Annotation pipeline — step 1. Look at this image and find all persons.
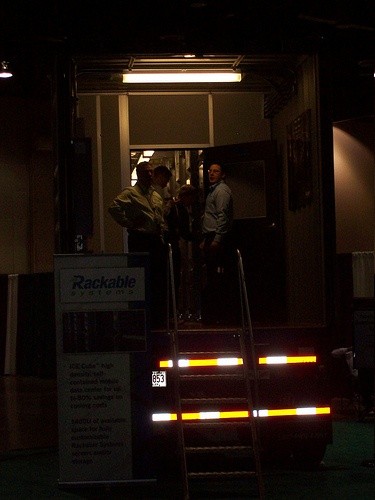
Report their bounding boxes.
[109,161,167,332]
[155,166,206,321]
[200,163,234,324]
